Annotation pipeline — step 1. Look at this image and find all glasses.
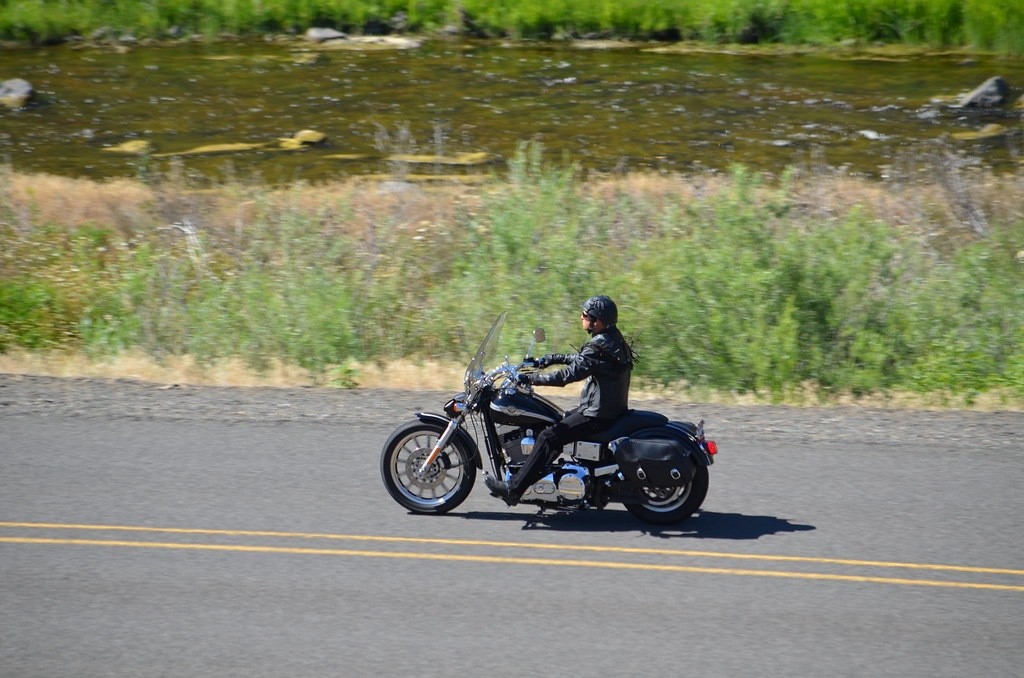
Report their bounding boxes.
[583,312,589,318]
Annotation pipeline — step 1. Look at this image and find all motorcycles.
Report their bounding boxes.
[380,310,719,525]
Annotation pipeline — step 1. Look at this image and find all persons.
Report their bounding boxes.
[483,296,632,505]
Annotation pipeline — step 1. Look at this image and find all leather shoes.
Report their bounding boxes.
[484,474,520,506]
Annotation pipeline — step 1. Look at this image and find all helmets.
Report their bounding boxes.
[583,295,617,324]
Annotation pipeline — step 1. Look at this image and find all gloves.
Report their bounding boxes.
[541,354,553,366]
[514,373,529,386]
